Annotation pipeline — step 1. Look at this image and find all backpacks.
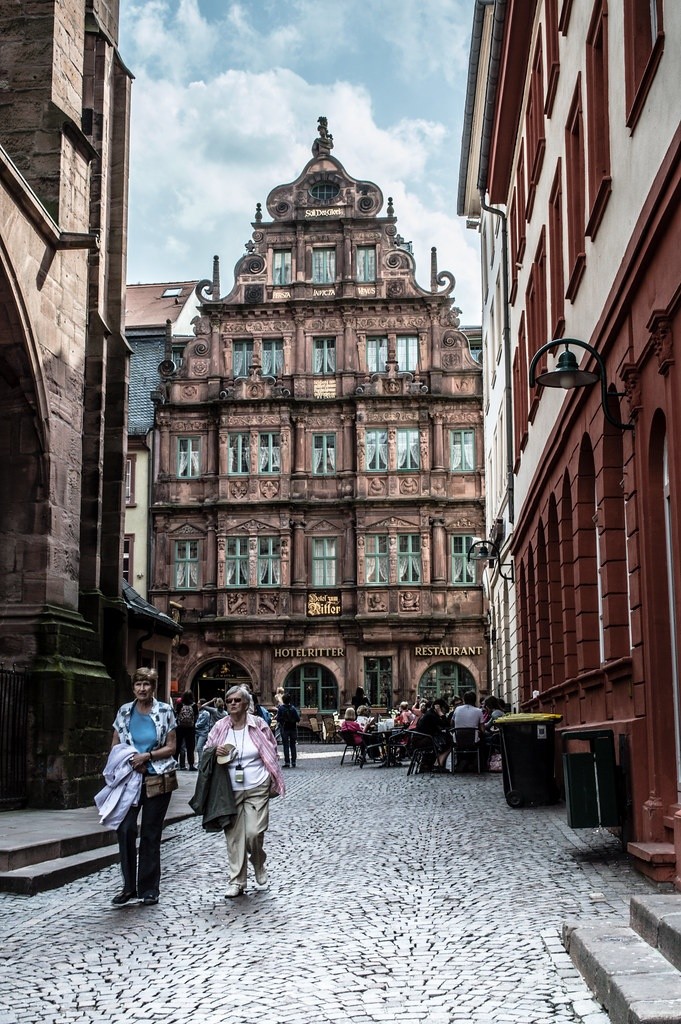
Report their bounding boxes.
[177,702,195,728]
[282,706,297,730]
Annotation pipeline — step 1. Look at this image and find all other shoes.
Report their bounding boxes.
[282,765,290,768]
[225,884,243,898]
[189,767,198,771]
[256,866,267,885]
[432,764,449,773]
[113,892,137,904]
[292,764,296,767]
[180,768,187,770]
[145,896,158,905]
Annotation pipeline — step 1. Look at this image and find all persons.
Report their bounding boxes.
[351,686,372,718]
[111,668,177,906]
[170,683,301,771]
[341,691,506,773]
[202,686,285,898]
[311,125,334,157]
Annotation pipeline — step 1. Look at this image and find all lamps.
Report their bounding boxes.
[467,541,514,584]
[529,337,634,431]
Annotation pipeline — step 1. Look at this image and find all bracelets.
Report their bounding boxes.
[146,751,152,762]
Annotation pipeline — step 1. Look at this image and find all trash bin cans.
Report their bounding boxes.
[493,713,564,809]
[560,728,622,829]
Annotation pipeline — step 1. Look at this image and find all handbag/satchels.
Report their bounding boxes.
[145,770,178,797]
[489,754,502,772]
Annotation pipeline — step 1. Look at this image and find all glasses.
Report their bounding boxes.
[226,698,241,703]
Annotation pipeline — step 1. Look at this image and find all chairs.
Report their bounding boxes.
[336,729,366,766]
[404,729,443,776]
[357,731,387,769]
[448,727,484,776]
[388,729,411,768]
